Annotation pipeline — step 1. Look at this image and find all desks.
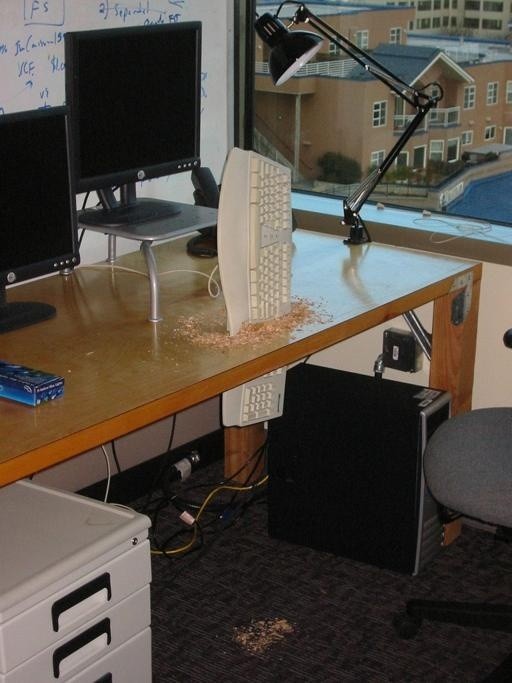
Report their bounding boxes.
[0,229,482,546]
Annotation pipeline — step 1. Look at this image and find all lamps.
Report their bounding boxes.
[254,0,444,244]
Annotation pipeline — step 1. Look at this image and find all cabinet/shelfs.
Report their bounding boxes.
[0,480,153,683]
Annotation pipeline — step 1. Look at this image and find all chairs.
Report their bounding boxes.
[391,329,512,683]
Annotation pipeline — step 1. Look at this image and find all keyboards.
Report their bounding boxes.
[216,146,293,431]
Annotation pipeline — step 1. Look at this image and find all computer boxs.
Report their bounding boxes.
[268,362,454,581]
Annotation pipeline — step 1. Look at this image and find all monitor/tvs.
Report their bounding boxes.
[1,103,82,337]
[61,19,203,229]
